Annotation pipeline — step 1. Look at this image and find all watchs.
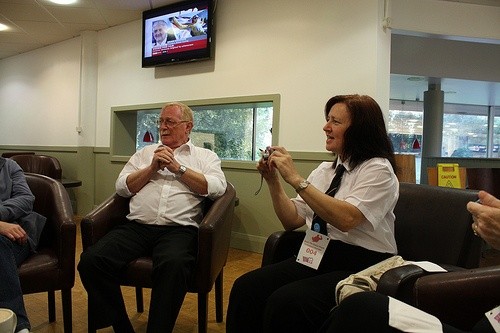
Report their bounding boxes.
[296,180,310,193]
[177,165,186,176]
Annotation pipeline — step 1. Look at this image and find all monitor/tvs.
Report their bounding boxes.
[141,0,215,68]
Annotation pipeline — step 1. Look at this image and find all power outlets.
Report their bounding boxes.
[76,127,82,132]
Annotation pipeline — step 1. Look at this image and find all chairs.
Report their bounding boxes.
[259,181,488,333]
[15,171,77,333]
[77,180,237,333]
[10,154,63,180]
[412,264,500,333]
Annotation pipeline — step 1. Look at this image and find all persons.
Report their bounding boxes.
[76,102,227,333]
[170,15,206,37]
[226,96,399,333]
[152,21,176,45]
[467,191,500,249]
[0,156,35,333]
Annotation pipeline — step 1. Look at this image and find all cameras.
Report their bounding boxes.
[262,148,275,160]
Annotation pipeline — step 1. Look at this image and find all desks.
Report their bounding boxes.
[54,178,82,188]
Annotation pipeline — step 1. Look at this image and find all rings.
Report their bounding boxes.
[474,226,478,235]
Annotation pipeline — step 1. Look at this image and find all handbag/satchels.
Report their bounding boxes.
[335,256,405,306]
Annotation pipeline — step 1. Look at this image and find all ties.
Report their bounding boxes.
[311,164,345,236]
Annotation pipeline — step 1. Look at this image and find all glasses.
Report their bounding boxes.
[156,119,189,129]
